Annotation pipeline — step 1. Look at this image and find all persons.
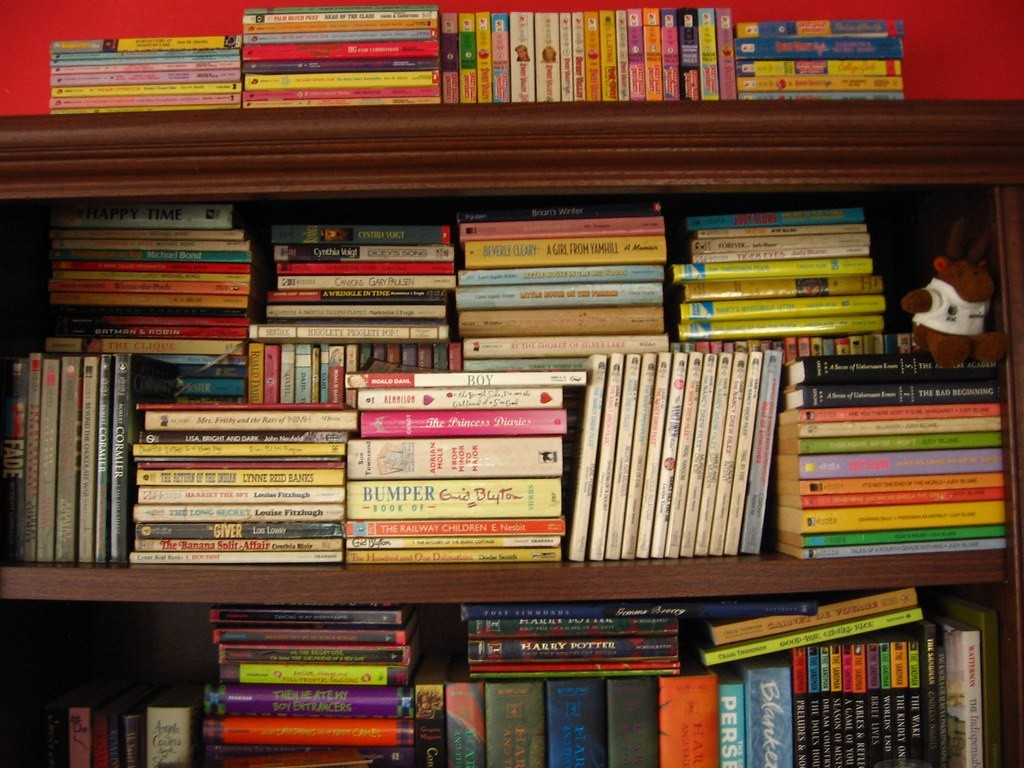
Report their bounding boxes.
[515,45,530,62]
[540,46,556,63]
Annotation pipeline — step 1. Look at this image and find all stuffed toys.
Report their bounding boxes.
[901,216,1010,368]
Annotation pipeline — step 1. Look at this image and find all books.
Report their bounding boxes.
[0,200,1010,565]
[40,585,1003,768]
[440,7,737,104]
[733,19,905,99]
[50,35,243,114]
[242,4,443,108]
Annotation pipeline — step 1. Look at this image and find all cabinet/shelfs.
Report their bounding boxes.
[1,99,1024,767]
